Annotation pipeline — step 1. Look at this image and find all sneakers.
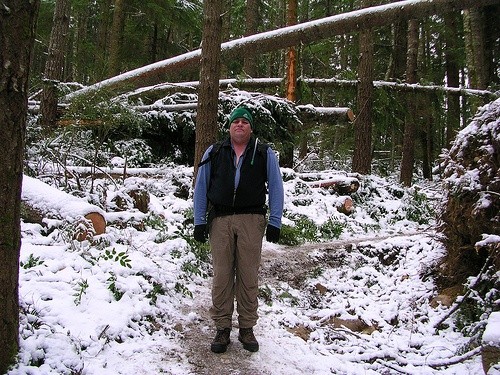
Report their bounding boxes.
[238,328,259,352]
[211,330,230,353]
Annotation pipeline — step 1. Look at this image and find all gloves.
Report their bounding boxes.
[266,224,280,243]
[193,224,208,243]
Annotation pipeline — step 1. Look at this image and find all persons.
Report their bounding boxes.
[191,107,284,352]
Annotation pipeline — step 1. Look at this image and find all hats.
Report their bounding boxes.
[229,108,253,129]
